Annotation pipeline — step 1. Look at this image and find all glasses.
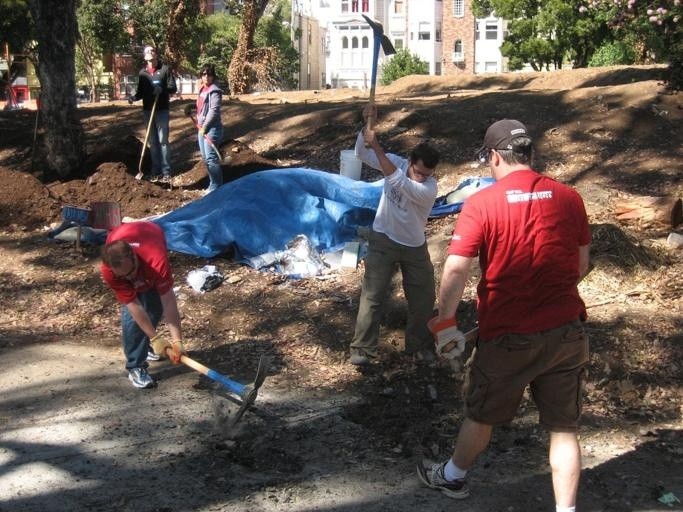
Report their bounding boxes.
[477,149,492,162]
[412,165,435,179]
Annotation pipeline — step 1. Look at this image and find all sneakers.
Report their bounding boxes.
[126,366,155,389]
[145,351,161,362]
[415,458,474,500]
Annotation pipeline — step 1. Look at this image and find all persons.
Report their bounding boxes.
[129,45,177,181]
[101,220,186,388]
[350,102,436,366]
[197,64,225,197]
[416,119,589,511]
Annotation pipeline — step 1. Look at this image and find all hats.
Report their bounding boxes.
[475,118,530,155]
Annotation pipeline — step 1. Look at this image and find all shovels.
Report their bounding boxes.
[135,92,159,180]
[188,113,232,165]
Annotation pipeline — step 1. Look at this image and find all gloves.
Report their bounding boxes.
[171,340,185,368]
[147,334,172,358]
[426,313,466,361]
